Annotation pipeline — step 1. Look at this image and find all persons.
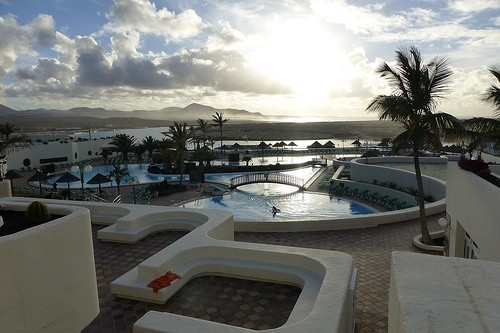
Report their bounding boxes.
[25,201,50,225]
[272,206,277,212]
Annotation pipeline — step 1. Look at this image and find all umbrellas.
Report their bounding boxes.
[56,172,81,192]
[377,142,389,151]
[26,170,51,195]
[256,141,297,156]
[86,172,113,198]
[220,143,241,153]
[352,140,361,147]
[309,140,335,153]
[2,170,23,196]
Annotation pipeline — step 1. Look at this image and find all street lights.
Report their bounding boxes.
[71,161,93,202]
